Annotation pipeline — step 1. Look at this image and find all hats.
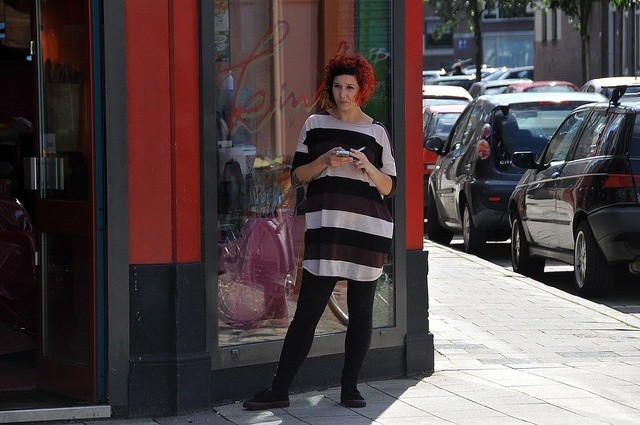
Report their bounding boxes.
[580,75,640,96]
[427,72,476,90]
[428,91,610,252]
[508,84,640,292]
[423,103,468,208]
[468,78,532,98]
[423,84,473,104]
[463,64,534,79]
[503,80,579,91]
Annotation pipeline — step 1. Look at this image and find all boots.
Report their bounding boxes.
[423,16,454,48]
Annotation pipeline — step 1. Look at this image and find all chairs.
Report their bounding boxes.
[341,385,367,407]
[243,387,290,409]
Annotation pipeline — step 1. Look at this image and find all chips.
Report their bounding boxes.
[361,168,366,173]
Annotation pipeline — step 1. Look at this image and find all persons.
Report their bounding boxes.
[243,53,399,411]
[452,63,466,75]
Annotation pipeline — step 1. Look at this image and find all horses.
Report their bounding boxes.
[336,151,350,156]
